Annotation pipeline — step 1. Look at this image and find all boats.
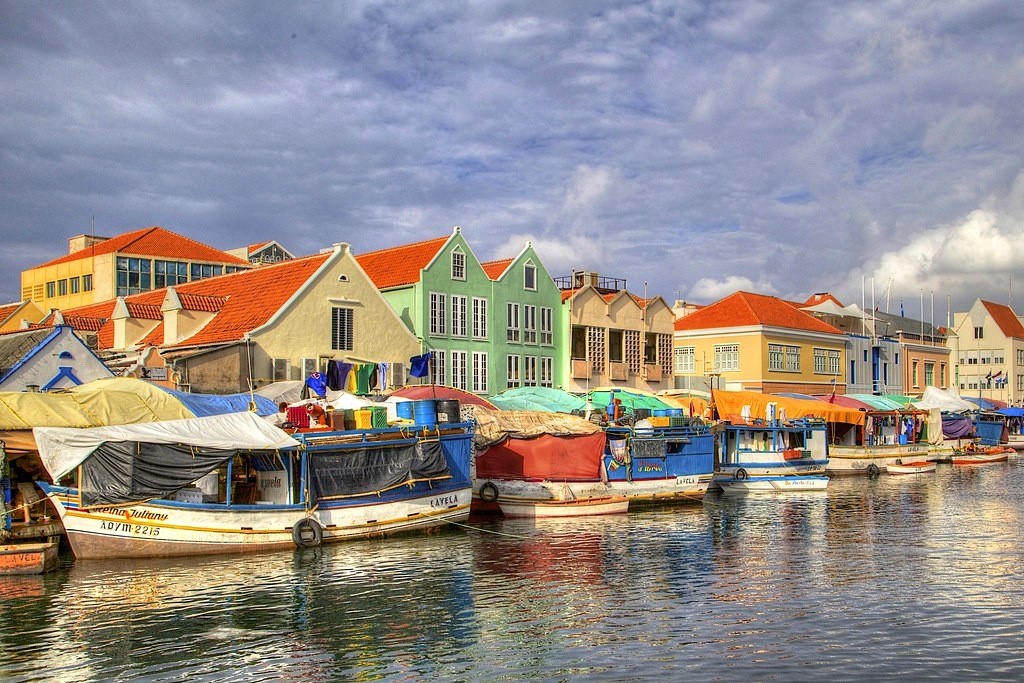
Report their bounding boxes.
[676,391,1024,482]
[951,449,1010,464]
[467,409,631,517]
[714,475,832,492]
[887,462,938,474]
[0,543,59,577]
[32,411,479,562]
[603,425,714,505]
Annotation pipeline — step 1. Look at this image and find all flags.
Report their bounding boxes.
[986,372,991,384]
[1003,372,1008,383]
[993,371,1001,383]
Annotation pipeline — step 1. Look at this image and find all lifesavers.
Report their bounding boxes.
[689,418,704,431]
[866,464,878,474]
[733,468,747,480]
[479,482,499,502]
[292,518,323,547]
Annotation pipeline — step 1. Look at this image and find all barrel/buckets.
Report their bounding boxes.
[899,435,907,444]
[414,399,437,429]
[397,401,414,419]
[633,408,651,423]
[437,399,460,429]
[654,410,666,417]
[666,408,683,416]
